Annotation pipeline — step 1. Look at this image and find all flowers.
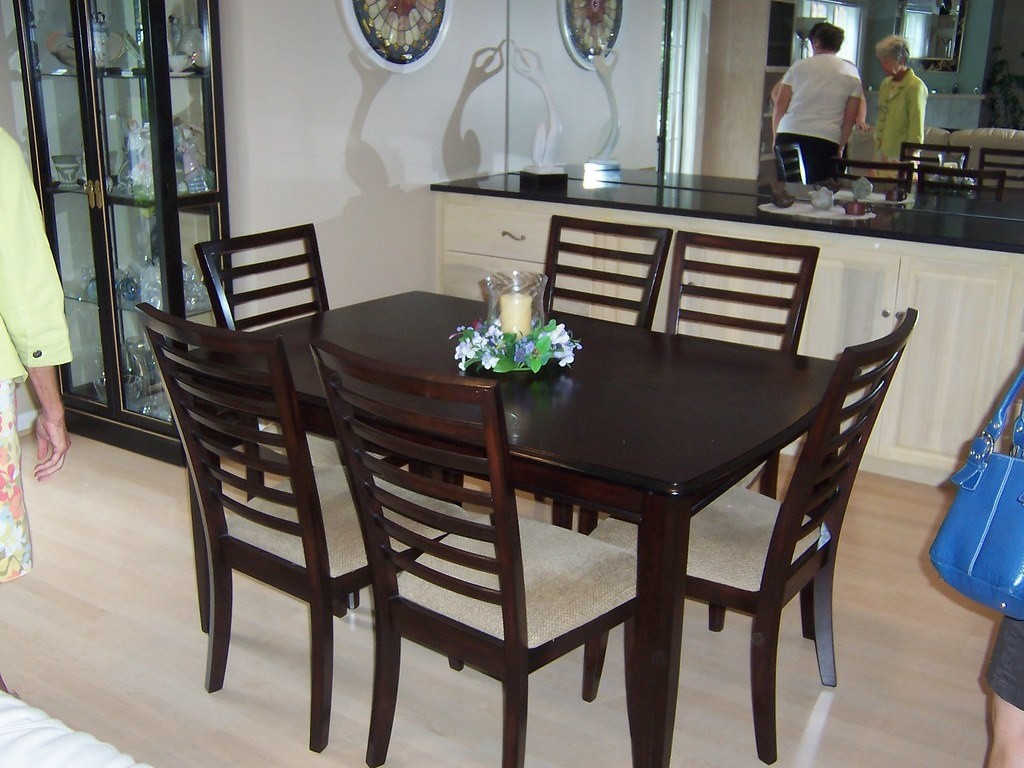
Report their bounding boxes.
[448,319,583,372]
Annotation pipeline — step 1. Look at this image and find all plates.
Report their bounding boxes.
[170,72,194,77]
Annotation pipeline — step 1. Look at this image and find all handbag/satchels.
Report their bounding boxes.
[927,370,1024,621]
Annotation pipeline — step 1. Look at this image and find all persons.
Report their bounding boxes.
[0,122,71,697]
[771,22,929,189]
[984,367,1024,768]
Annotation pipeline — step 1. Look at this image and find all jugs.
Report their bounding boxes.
[91,341,147,410]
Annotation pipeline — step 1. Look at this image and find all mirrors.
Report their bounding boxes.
[656,0,1024,220]
[892,0,969,73]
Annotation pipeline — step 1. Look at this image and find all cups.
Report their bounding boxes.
[937,153,966,183]
[168,54,189,71]
[479,270,548,337]
[51,153,81,190]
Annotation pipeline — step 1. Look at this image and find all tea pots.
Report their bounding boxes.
[840,198,874,216]
[851,175,873,198]
[808,187,834,210]
[883,187,907,201]
[813,178,844,195]
[770,190,796,208]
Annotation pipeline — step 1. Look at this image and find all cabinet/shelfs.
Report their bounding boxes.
[13,0,233,467]
[431,193,1022,488]
[700,0,795,180]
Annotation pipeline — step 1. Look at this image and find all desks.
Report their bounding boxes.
[193,291,838,768]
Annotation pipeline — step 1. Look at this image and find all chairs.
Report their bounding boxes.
[774,139,1024,202]
[134,212,919,768]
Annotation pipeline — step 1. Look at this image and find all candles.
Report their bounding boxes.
[943,163,958,179]
[500,293,532,341]
